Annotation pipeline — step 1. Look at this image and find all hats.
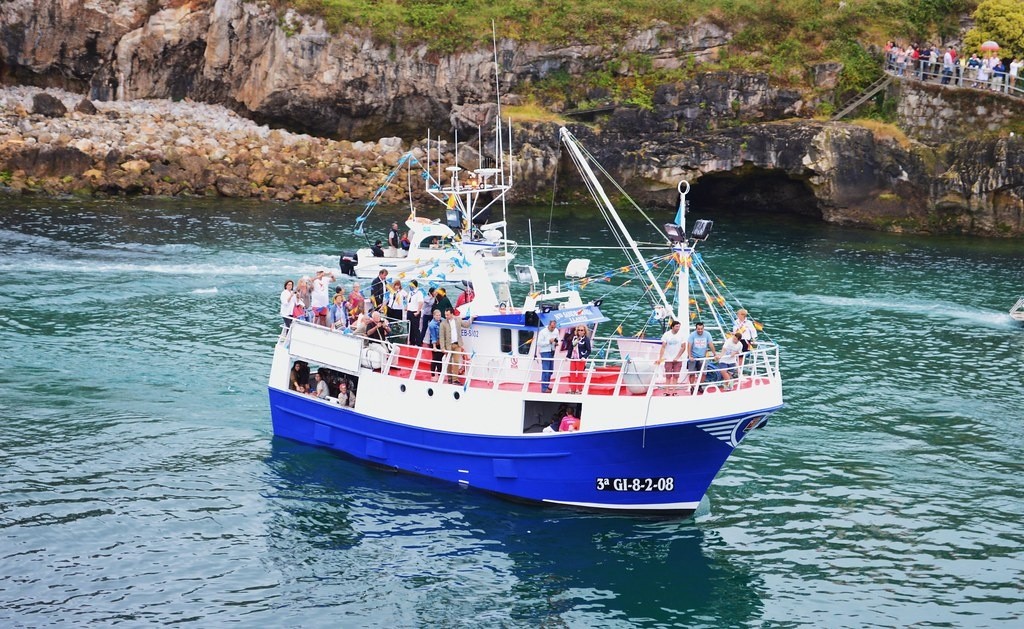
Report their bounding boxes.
[317,267,324,272]
[353,282,360,287]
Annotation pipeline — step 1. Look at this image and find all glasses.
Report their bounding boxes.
[578,330,584,331]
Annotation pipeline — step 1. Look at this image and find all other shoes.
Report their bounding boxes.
[672,393,679,396]
[690,387,694,393]
[453,380,462,385]
[542,390,551,393]
[663,393,670,396]
[699,386,704,392]
[548,387,552,390]
[448,378,453,383]
[726,383,733,391]
[431,377,438,381]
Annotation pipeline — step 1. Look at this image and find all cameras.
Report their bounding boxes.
[554,337,560,343]
[293,291,297,294]
[379,320,384,324]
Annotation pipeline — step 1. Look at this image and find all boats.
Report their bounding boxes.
[267,17,786,519]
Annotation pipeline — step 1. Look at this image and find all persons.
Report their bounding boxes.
[885,40,1024,94]
[566,324,591,394]
[290,361,356,408]
[732,310,757,378]
[655,321,686,396]
[548,406,580,432]
[439,308,478,385]
[430,288,453,321]
[429,310,445,381]
[688,322,719,394]
[401,232,410,250]
[339,254,354,275]
[388,223,399,249]
[454,286,475,308]
[538,320,559,393]
[428,238,440,248]
[280,269,438,370]
[372,241,384,257]
[720,333,743,390]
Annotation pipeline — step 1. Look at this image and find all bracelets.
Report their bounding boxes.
[714,354,716,356]
[386,326,388,329]
[331,276,334,278]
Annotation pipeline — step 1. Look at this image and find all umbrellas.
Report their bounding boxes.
[980,41,1000,56]
